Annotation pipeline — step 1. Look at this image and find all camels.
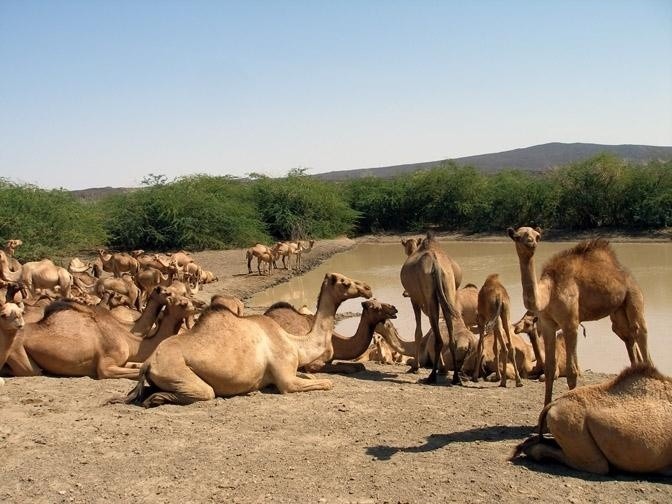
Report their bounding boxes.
[0,229,568,388]
